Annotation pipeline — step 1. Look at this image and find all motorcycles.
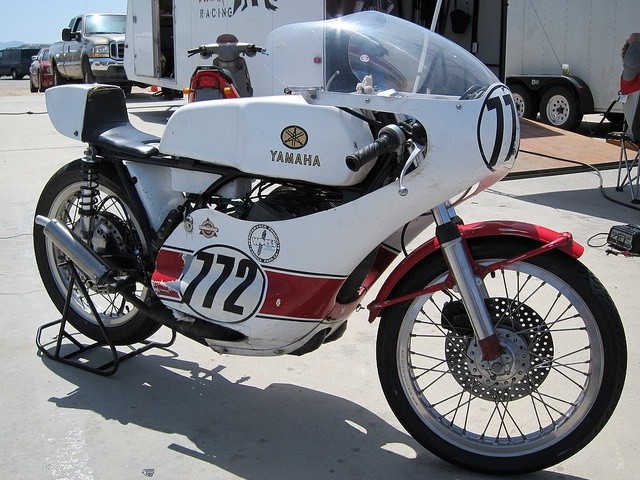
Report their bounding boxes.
[182,33,269,102]
[29,5,627,474]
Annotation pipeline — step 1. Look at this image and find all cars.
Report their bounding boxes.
[29,47,54,93]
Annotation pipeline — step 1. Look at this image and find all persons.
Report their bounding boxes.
[618,33,640,149]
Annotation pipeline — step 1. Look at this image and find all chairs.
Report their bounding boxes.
[616,90,640,203]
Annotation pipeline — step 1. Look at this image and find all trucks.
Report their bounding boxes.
[504,0,640,129]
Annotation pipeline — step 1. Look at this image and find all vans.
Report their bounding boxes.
[0,47,41,80]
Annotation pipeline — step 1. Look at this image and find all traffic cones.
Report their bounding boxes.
[148,84,158,92]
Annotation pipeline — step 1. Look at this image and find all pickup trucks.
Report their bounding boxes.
[48,12,150,93]
